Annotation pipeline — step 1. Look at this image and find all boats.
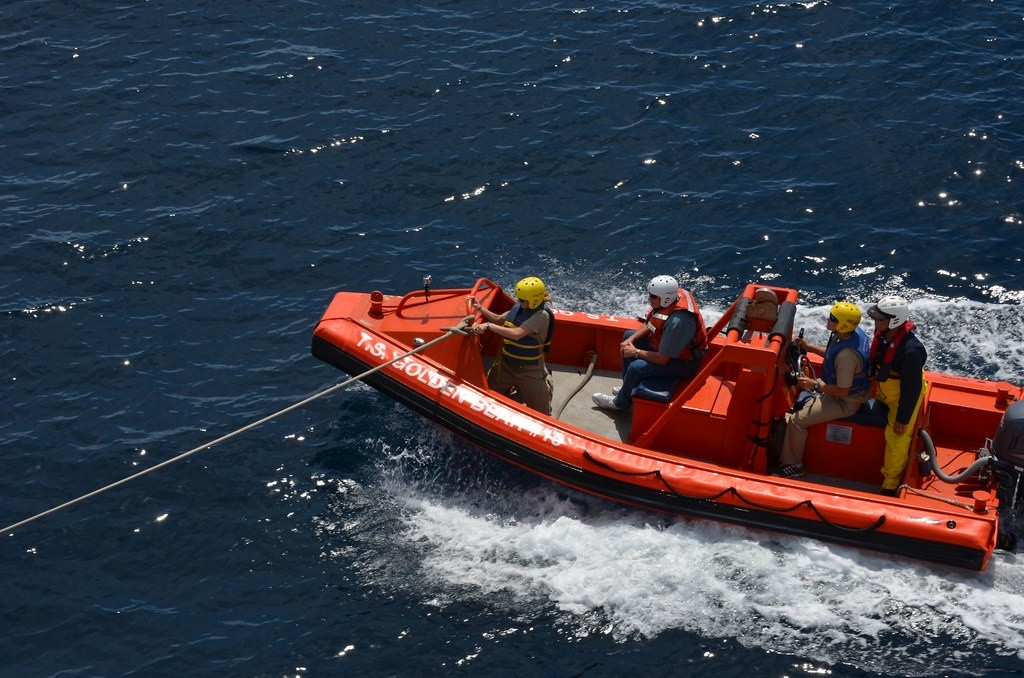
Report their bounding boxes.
[310,277,1024,571]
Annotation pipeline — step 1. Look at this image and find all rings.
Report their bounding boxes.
[804,381,806,384]
[900,428,902,431]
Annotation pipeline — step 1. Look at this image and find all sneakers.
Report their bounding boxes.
[592,392,624,410]
[772,462,805,478]
[611,386,622,396]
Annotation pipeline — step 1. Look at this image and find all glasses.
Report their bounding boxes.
[517,298,525,303]
[649,293,659,299]
[830,313,839,324]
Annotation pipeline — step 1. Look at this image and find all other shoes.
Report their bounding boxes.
[881,488,896,497]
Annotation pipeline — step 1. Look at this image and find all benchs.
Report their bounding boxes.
[794,390,891,486]
[632,367,724,457]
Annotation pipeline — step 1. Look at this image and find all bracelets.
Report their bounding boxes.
[486,322,489,330]
[478,305,482,310]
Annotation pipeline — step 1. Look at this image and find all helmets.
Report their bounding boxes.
[514,277,545,309]
[647,275,678,307]
[830,302,862,333]
[867,296,909,329]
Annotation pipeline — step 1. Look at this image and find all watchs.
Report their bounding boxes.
[634,349,640,359]
[814,381,820,389]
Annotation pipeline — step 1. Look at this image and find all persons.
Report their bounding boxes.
[771,301,873,478]
[866,295,928,496]
[592,275,709,411]
[465,276,556,416]
